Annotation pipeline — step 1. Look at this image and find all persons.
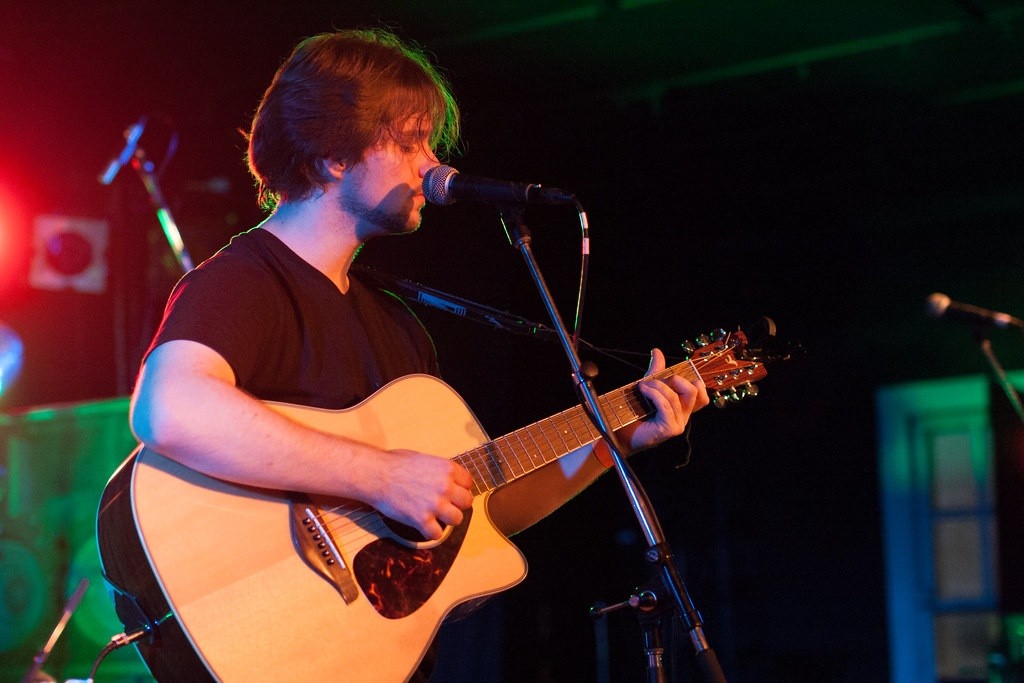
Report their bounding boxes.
[130,32,709,683]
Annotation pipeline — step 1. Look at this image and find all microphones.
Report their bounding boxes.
[422,165,577,206]
[99,122,150,186]
[924,293,1024,330]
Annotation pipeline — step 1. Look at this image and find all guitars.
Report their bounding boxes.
[93,322,786,683]
